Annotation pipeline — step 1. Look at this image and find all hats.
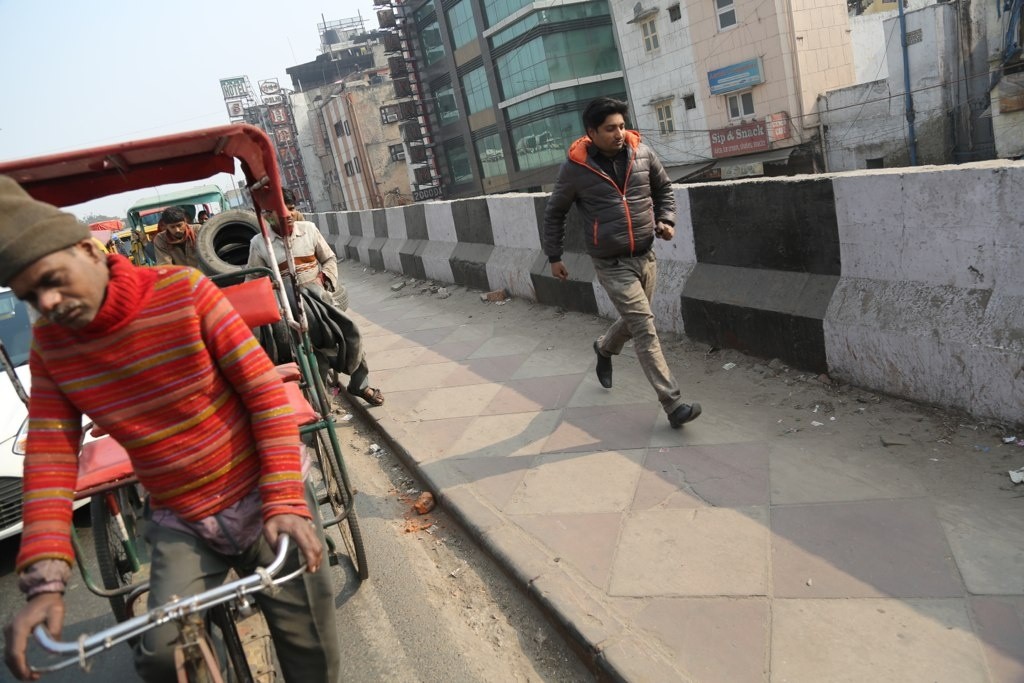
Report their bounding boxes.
[0,175,91,287]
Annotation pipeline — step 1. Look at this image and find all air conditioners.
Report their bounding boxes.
[397,152,405,160]
[387,113,399,122]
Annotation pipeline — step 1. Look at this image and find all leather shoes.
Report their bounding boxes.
[669,399,701,428]
[593,340,613,388]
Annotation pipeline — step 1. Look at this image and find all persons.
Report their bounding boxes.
[542,95,702,431]
[243,187,386,407]
[153,207,210,269]
[0,175,342,683]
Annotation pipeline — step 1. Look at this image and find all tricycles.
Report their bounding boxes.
[1,123,370,683]
[126,185,228,271]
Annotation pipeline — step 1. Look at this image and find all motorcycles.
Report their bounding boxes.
[85,219,135,264]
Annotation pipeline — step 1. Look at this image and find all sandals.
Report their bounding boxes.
[347,383,384,406]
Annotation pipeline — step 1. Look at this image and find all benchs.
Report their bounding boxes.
[55,277,327,499]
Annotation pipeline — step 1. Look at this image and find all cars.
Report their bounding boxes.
[0,284,110,569]
